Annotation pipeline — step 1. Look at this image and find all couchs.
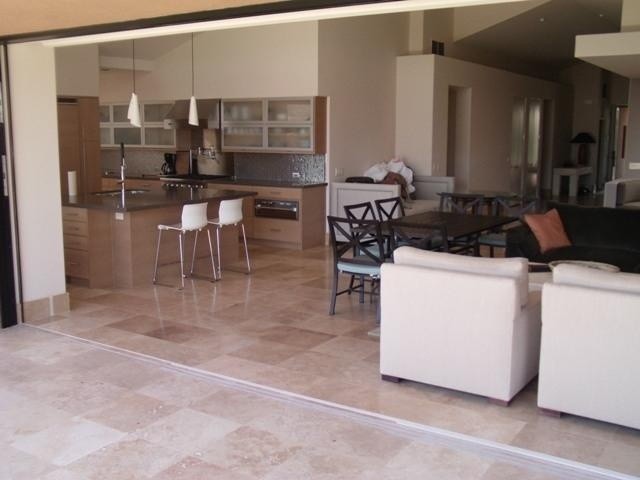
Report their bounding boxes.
[325,175,455,247]
[505,201,640,274]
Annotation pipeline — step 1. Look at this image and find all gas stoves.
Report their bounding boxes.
[159,172,231,189]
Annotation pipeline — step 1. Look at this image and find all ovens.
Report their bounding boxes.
[254,199,299,220]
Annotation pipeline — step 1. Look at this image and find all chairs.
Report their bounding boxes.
[379,246,542,407]
[188,197,250,282]
[326,192,541,324]
[152,202,218,289]
[536,263,639,430]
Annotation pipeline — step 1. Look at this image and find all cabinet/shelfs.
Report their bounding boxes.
[254,187,325,249]
[99,96,191,150]
[221,96,326,155]
[61,206,89,286]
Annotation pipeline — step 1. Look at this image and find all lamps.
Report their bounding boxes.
[570,132,596,167]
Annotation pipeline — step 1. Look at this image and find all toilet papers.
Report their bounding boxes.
[67,171,78,196]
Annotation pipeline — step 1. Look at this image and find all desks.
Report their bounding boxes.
[552,167,598,205]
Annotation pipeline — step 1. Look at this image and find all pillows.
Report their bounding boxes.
[523,208,572,254]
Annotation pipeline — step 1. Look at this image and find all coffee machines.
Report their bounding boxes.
[161,153,176,174]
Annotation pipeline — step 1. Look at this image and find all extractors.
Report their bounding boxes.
[165,99,221,120]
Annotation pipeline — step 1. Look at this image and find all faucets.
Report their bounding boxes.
[116,196,125,209]
[117,166,125,189]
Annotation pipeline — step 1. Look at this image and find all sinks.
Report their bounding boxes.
[95,189,151,197]
